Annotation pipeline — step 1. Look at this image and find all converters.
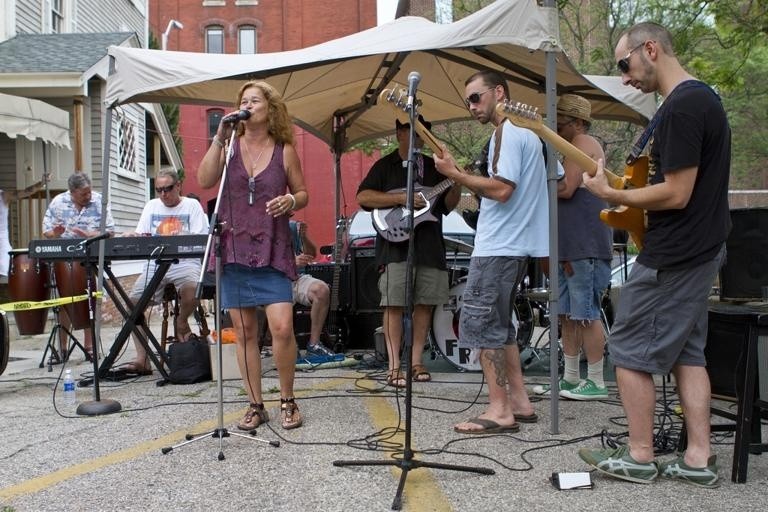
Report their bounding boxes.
[354,354,361,360]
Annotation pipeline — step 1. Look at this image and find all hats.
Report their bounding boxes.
[395,112,434,131]
[556,92,595,126]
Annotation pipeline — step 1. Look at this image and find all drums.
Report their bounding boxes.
[8,249,52,335]
[55,260,97,330]
[432,273,536,372]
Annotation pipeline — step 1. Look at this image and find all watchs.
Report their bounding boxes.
[213,135,226,148]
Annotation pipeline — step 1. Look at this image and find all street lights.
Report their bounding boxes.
[161,20,183,50]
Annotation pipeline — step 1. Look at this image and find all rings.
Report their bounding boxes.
[278,203,281,207]
[283,210,286,213]
[280,206,284,210]
[275,198,280,202]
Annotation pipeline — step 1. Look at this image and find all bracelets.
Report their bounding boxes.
[286,193,297,210]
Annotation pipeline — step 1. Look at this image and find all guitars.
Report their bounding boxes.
[495,98,649,251]
[371,148,488,243]
[379,82,484,222]
[321,214,351,354]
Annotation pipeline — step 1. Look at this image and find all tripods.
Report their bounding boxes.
[38,260,93,372]
[331,113,496,510]
[161,119,281,460]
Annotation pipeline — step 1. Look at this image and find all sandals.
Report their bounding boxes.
[278,399,303,429]
[81,347,103,359]
[45,344,70,365]
[235,403,270,432]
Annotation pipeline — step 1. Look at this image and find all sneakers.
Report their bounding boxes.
[306,340,336,358]
[578,444,660,484]
[656,448,720,488]
[558,377,611,402]
[531,378,580,395]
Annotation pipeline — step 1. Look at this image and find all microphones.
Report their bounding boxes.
[78,232,110,246]
[407,71,421,108]
[223,110,251,123]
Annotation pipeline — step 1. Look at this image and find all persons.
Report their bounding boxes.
[432,70,551,433]
[195,79,311,432]
[356,116,463,388]
[534,94,614,400]
[41,171,116,363]
[576,21,732,490]
[120,166,211,376]
[287,212,346,370]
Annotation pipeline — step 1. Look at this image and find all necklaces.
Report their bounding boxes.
[243,135,270,207]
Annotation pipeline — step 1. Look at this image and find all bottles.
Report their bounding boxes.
[63,370,75,412]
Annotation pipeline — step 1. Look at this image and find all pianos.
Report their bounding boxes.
[28,233,214,260]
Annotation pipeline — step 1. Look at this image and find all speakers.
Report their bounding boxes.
[702,307,760,399]
[348,244,387,310]
[719,207,768,302]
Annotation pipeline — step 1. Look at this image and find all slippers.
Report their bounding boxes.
[512,409,539,424]
[408,361,433,384]
[386,365,408,389]
[114,362,153,377]
[454,410,521,435]
[172,317,193,342]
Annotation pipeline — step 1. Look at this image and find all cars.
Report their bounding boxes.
[319,209,476,262]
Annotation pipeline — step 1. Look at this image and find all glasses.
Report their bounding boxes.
[462,86,494,103]
[155,182,176,192]
[608,40,661,75]
